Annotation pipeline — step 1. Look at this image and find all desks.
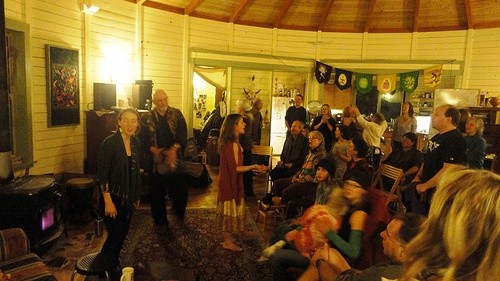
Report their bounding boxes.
[256,199,286,231]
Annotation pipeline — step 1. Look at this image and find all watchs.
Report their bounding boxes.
[172,144,177,148]
[315,258,325,267]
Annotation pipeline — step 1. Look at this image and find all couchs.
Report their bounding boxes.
[0,228,57,281]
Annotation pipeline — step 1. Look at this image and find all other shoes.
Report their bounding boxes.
[260,245,279,258]
[275,215,283,222]
[222,243,243,252]
[174,208,186,220]
[276,207,281,214]
[255,254,273,266]
[153,213,170,226]
[237,230,256,240]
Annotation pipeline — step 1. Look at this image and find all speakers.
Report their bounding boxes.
[132,84,152,109]
[93,82,117,111]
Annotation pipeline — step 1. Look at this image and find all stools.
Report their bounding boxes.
[61,178,100,237]
[71,252,110,281]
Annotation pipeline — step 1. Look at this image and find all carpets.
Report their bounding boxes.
[117,207,273,281]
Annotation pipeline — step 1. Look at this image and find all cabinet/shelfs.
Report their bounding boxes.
[469,107,500,175]
[0,173,64,256]
[85,109,149,173]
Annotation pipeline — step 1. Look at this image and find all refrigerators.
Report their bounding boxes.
[270,96,295,155]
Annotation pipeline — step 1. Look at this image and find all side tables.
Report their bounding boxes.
[413,97,434,111]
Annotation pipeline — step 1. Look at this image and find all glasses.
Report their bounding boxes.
[320,107,328,111]
[308,136,319,140]
[343,181,364,190]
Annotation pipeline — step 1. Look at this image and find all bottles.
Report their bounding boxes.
[279,88,300,97]
[95,216,104,237]
[489,96,500,108]
[485,91,489,107]
[144,99,152,110]
[480,91,485,106]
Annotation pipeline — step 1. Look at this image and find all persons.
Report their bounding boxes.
[236,97,263,203]
[218,113,264,251]
[263,120,328,211]
[89,107,142,281]
[329,125,353,179]
[390,102,417,152]
[339,105,387,171]
[403,104,486,219]
[298,169,500,281]
[284,94,306,139]
[311,104,336,153]
[258,157,375,281]
[140,89,187,237]
[379,133,423,197]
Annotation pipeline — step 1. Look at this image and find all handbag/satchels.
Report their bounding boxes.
[260,193,273,211]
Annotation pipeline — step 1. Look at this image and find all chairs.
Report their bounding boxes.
[372,164,403,207]
[250,145,273,194]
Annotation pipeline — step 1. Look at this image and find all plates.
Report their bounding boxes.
[110,106,124,110]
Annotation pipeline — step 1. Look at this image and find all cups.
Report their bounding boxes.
[272,196,282,205]
[418,91,435,108]
[119,266,135,281]
[118,100,124,107]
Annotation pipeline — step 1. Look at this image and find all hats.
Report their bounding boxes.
[314,156,337,179]
[343,160,374,189]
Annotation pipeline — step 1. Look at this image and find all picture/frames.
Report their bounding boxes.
[45,44,81,127]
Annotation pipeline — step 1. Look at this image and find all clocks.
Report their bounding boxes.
[307,101,322,114]
